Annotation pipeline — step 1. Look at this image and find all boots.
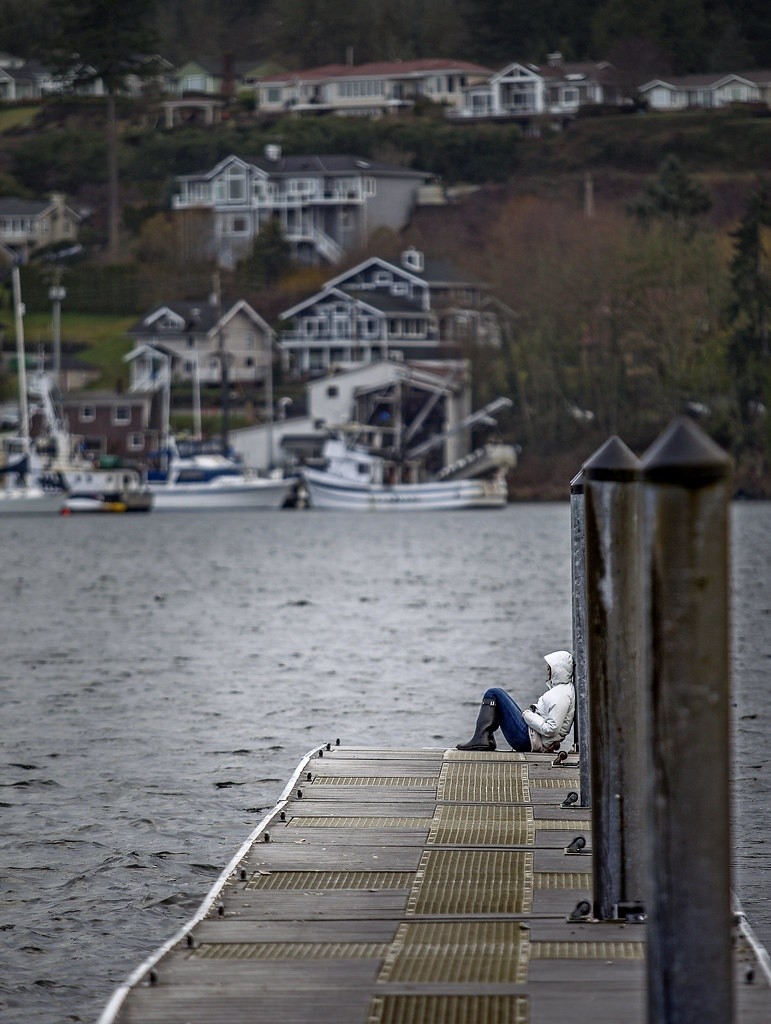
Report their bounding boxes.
[458,699,498,750]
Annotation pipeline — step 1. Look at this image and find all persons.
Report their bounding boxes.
[456,651,576,753]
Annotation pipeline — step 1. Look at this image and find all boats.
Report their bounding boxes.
[125,325,300,510]
[1,265,153,514]
[295,354,515,513]
